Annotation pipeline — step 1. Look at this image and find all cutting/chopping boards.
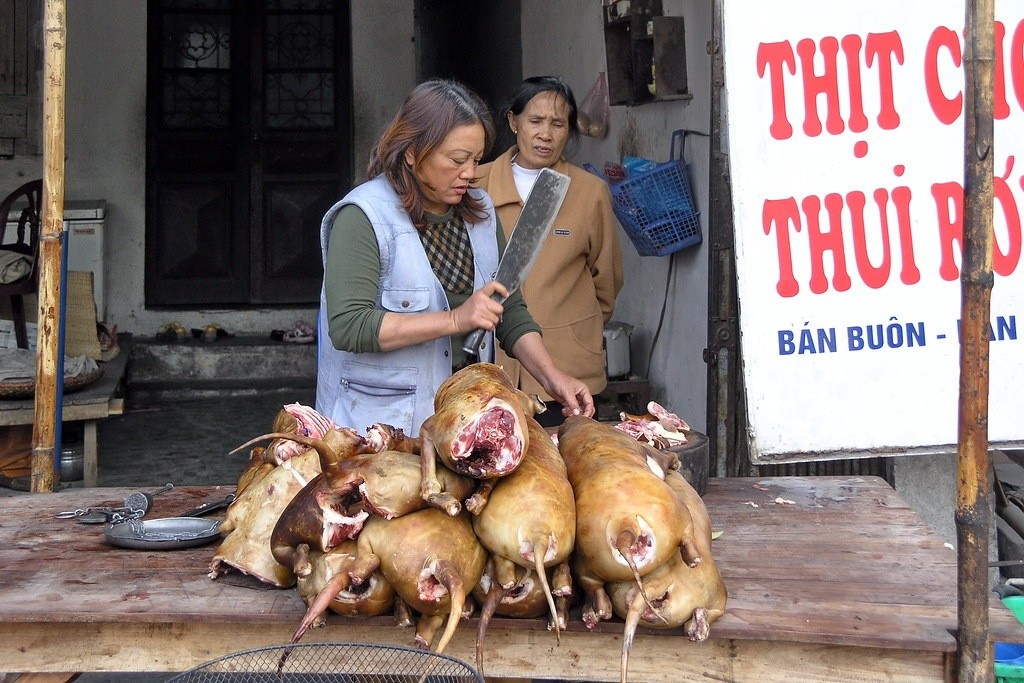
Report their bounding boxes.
[544,421,709,496]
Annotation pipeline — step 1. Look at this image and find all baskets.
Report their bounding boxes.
[583,131,703,256]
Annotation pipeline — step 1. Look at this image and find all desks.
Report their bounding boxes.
[0,476,1024,683]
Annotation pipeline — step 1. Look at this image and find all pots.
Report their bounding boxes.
[60,443,84,482]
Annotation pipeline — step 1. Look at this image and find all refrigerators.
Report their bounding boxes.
[0,200,108,321]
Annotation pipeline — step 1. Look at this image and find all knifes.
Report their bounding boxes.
[461,168,571,355]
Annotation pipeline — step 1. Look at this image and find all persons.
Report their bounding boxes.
[315,77,596,439]
[468,75,626,428]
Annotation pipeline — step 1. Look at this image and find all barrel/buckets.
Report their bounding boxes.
[994,596,1024,683]
